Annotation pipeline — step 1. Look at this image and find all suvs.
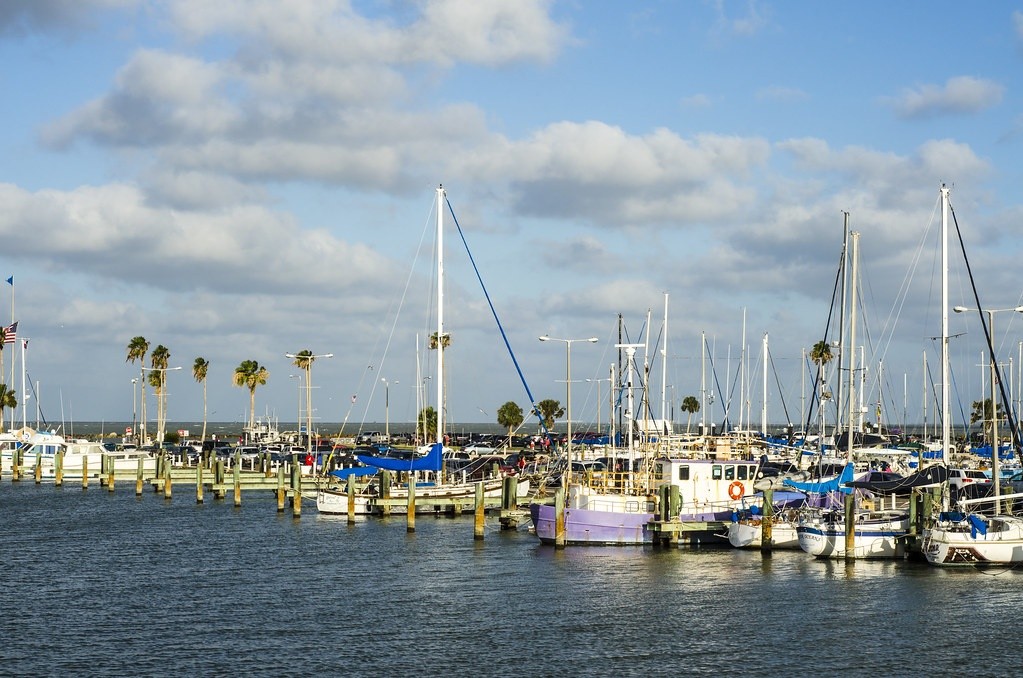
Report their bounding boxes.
[948,468,989,490]
[201,441,233,460]
[183,440,203,453]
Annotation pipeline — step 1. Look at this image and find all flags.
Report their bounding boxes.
[2,322,18,343]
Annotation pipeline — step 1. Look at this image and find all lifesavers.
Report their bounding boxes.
[728,481,745,500]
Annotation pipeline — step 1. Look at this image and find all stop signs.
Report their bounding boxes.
[125,427,133,432]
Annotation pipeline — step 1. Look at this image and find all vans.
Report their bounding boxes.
[65,439,88,443]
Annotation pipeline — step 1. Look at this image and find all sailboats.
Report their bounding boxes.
[316,178,1023,576]
[0,274,170,478]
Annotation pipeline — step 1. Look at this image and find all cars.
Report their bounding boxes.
[261,432,607,470]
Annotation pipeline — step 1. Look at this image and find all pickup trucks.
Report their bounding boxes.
[230,446,260,465]
[356,430,392,445]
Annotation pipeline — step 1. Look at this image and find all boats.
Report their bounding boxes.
[234,404,319,447]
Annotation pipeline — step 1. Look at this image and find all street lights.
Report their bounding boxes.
[538,336,598,485]
[382,378,399,442]
[142,366,182,448]
[952,306,1023,516]
[284,353,334,455]
